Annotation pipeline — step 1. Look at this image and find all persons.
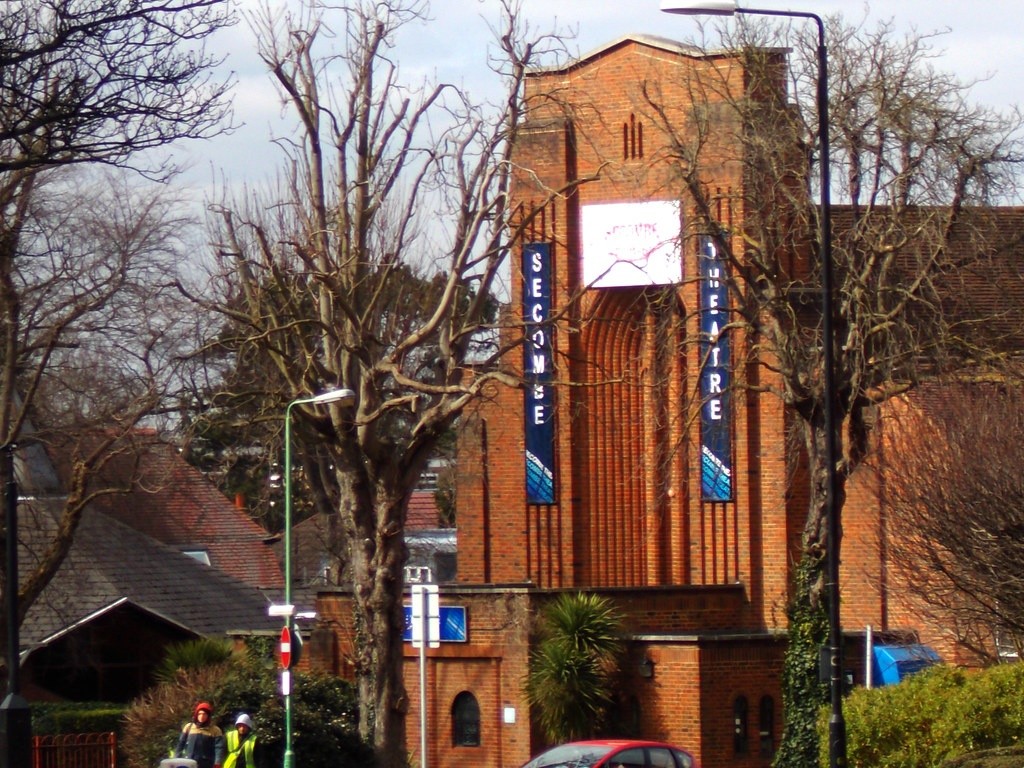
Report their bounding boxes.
[221,713,263,768]
[174,701,224,768]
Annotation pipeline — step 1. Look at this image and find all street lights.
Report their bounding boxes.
[283,389,354,768]
[662,1,849,768]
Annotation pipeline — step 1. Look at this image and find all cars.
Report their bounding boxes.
[518,739,697,768]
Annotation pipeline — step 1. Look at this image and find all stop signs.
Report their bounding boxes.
[280,626,292,669]
